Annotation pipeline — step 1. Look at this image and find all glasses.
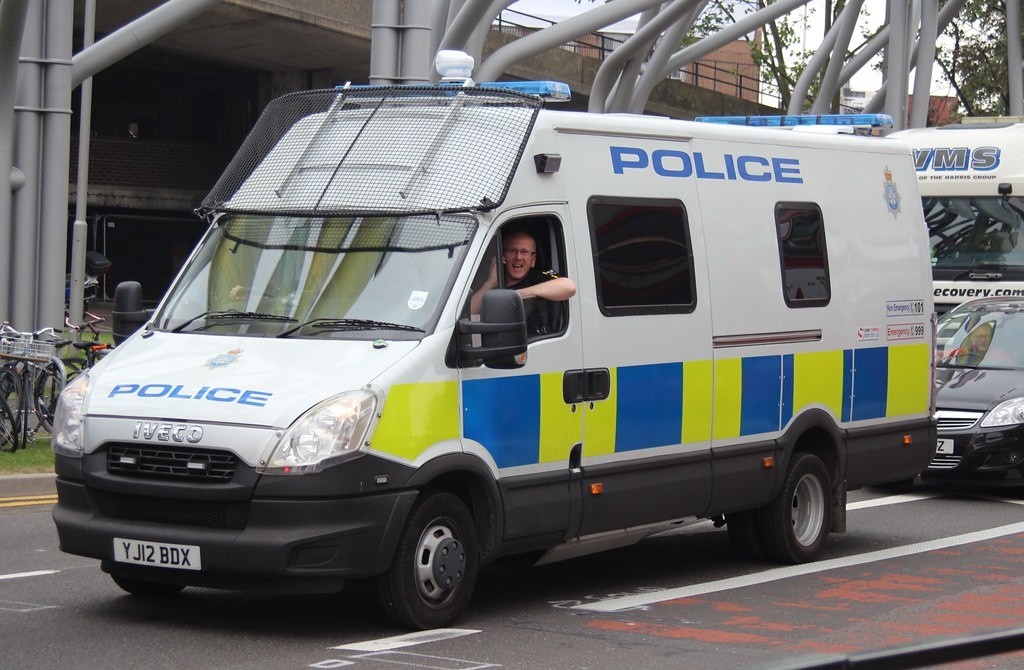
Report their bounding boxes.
[505,247,537,257]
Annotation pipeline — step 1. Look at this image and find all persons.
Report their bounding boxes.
[935,321,995,366]
[463,230,576,338]
[230,284,272,304]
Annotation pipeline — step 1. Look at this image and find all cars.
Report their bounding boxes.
[875,297,1024,493]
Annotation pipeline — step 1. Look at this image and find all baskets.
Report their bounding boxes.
[0,337,56,362]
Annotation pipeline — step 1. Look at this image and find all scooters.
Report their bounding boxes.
[65,251,112,310]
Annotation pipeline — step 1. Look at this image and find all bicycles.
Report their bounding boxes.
[0,310,122,449]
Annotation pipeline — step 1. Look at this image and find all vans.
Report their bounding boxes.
[874,113,1024,328]
[52,50,946,633]
